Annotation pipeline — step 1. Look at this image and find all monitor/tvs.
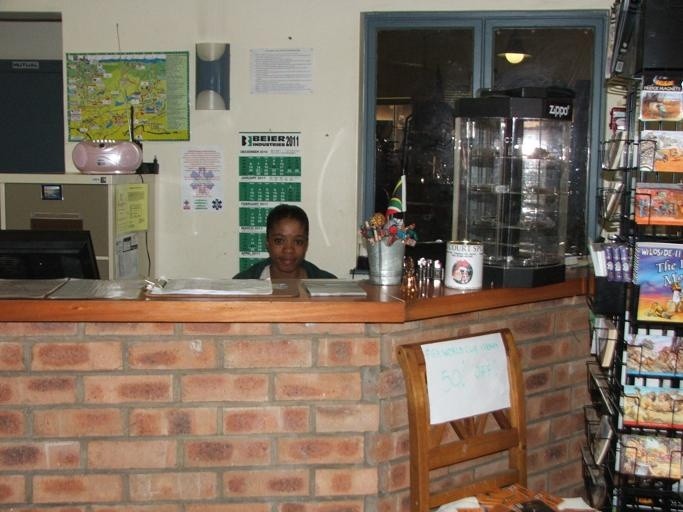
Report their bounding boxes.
[0,230,100,279]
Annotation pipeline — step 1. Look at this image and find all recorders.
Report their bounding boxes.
[72,139,143,175]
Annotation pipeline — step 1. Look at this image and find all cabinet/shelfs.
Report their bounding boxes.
[577,1,683,511]
[451,97,573,290]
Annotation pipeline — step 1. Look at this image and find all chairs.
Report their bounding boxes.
[395,327,601,511]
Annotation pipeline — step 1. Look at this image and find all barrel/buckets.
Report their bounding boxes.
[365,234,405,286]
[442,241,484,289]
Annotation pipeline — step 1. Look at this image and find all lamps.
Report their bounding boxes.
[195,43,230,110]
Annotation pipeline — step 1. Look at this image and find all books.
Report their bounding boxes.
[301,279,367,297]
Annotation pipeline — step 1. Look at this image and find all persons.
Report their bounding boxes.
[231,204,338,281]
